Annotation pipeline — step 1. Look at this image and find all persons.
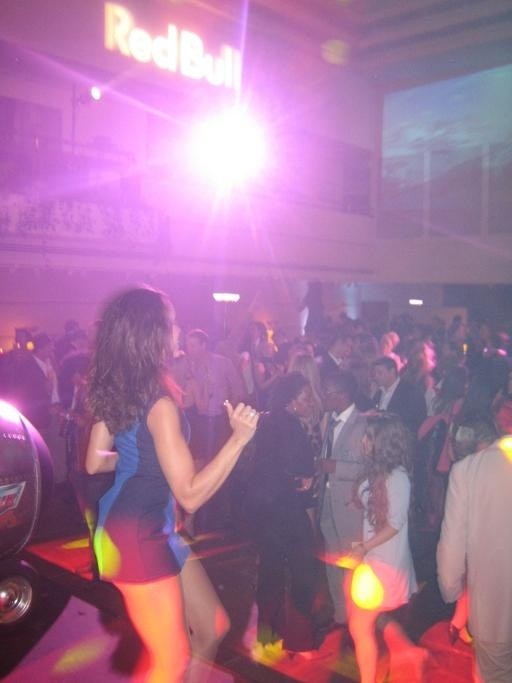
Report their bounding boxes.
[83,287,261,681]
[0,310,512,682]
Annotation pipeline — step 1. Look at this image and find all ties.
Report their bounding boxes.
[322,418,341,490]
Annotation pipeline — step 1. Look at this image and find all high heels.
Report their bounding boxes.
[283,645,332,662]
[447,622,475,645]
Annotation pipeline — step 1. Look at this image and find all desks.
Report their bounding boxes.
[25,526,262,621]
